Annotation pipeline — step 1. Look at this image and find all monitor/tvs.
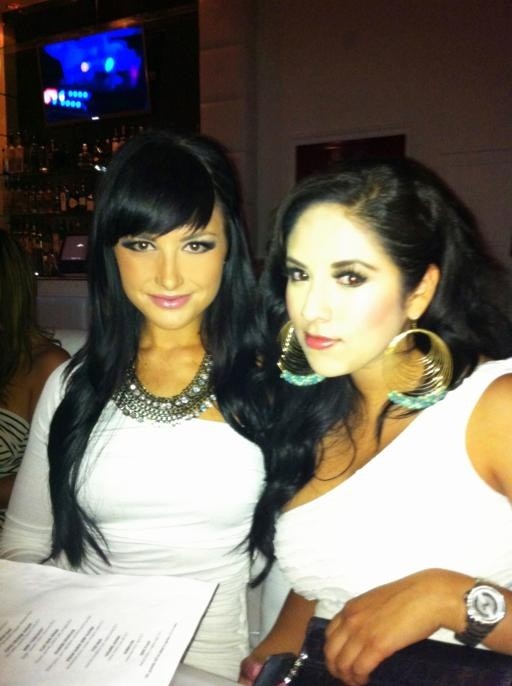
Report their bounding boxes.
[35,17,151,129]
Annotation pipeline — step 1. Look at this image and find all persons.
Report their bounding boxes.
[0,226,73,537]
[245,156,511,684]
[1,125,273,678]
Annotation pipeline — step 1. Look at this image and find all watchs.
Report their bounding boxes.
[453,579,506,648]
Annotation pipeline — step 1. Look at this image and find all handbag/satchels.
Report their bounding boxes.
[252,618,512,685]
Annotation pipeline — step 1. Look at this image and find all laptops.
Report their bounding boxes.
[61,235,89,262]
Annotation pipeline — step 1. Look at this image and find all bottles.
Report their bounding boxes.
[1,114,145,277]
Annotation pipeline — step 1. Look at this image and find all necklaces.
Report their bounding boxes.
[110,351,219,426]
